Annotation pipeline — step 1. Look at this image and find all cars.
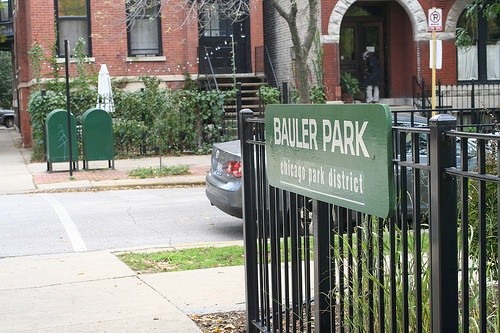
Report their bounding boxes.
[0,108,15,128]
[205,115,495,235]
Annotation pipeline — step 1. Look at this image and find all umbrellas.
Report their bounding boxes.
[96,63,115,114]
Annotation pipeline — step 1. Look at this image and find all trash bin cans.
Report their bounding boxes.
[44,109,79,173]
[82,108,114,171]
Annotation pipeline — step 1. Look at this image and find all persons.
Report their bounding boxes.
[361,45,379,103]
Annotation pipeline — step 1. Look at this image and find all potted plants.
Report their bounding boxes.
[340,72,360,103]
